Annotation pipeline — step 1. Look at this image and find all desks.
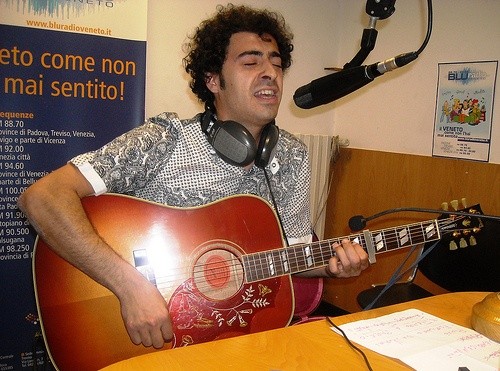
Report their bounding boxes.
[97,290,500,371]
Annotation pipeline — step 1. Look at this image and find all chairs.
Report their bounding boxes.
[357,203,500,311]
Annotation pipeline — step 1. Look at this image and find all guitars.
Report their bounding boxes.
[33,193,488,371]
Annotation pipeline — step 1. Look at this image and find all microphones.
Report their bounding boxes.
[293,51,419,109]
[349,207,500,232]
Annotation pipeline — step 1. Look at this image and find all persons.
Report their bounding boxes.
[17,5,370,349]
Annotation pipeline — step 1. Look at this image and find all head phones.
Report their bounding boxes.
[200,109,279,169]
